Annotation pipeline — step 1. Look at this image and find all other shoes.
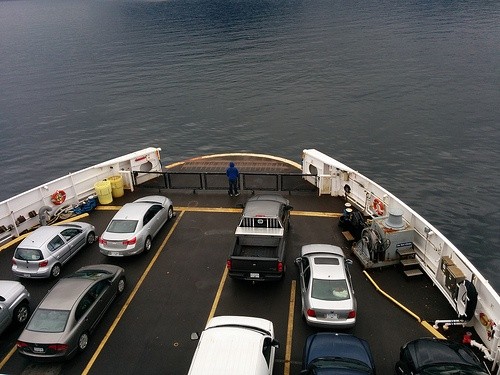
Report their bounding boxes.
[229,193,232,196]
[235,192,240,196]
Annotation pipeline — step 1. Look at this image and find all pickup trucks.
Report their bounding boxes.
[226,194,293,285]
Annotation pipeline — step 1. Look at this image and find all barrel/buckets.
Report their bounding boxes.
[94,175,124,204]
[345,203,353,219]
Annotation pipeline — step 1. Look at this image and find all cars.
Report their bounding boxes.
[17,264,126,361]
[11,223,96,281]
[393,336,491,375]
[188,315,281,375]
[298,331,375,375]
[99,195,174,258]
[293,244,358,328]
[0,280,32,336]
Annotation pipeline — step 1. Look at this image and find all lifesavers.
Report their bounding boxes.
[373,199,385,216]
[50,190,67,206]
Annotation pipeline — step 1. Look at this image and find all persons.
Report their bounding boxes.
[226,162,239,197]
